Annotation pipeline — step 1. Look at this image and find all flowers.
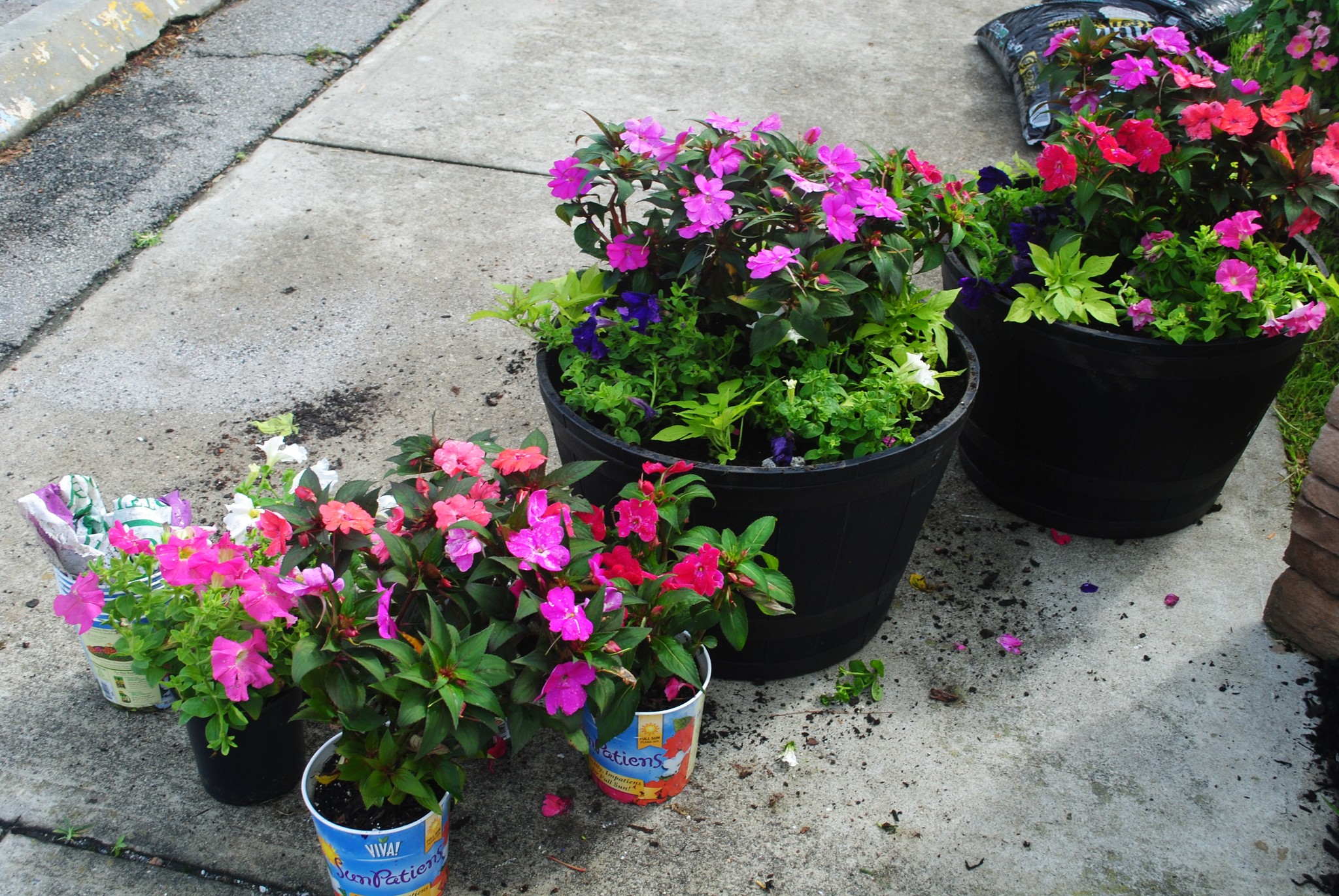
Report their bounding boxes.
[980,30,1339,346]
[507,111,978,446]
[53,430,803,805]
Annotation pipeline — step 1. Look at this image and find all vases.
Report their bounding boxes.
[51,563,184,713]
[176,672,295,811]
[529,303,974,682]
[297,728,452,896]
[964,265,1306,541]
[569,630,712,805]
